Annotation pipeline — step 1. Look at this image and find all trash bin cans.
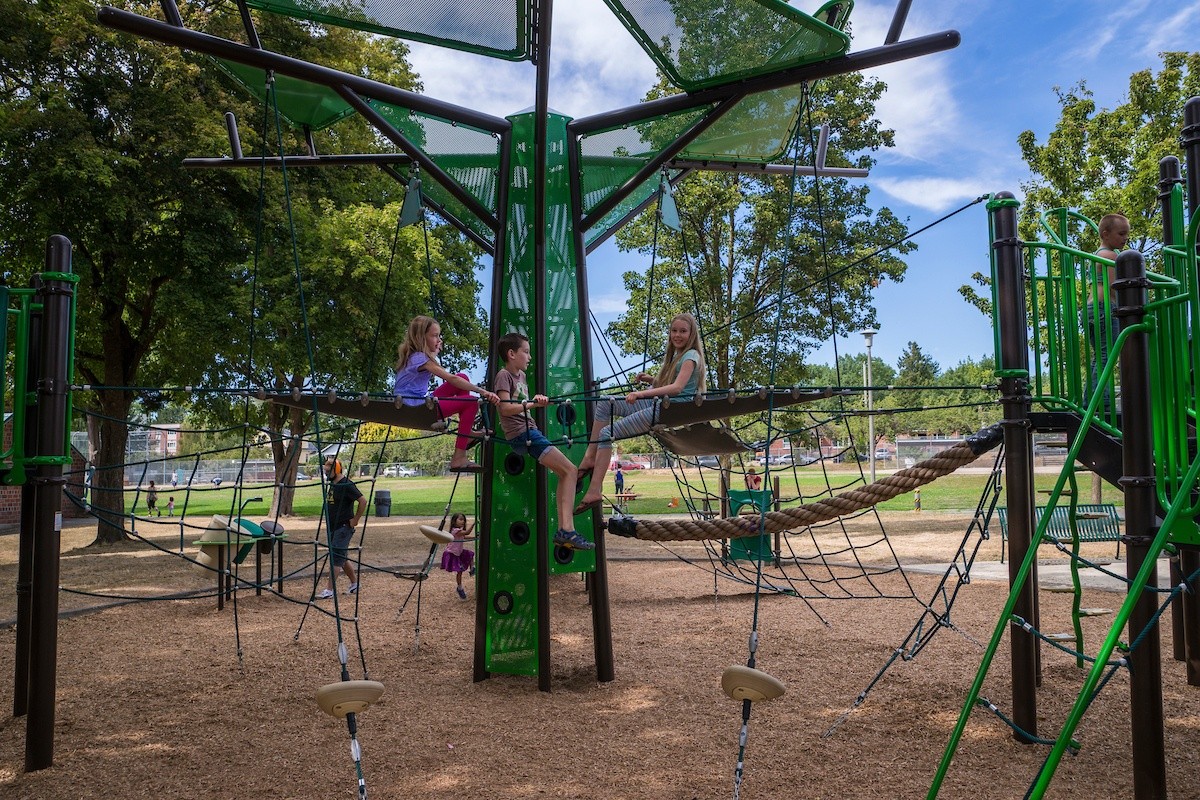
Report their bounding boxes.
[374,490,392,517]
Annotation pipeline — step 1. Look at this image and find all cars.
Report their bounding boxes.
[296,471,313,480]
[611,459,644,470]
[755,448,891,466]
[687,453,734,468]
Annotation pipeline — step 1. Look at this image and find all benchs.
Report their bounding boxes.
[997,503,1125,563]
[696,509,760,520]
[603,504,628,518]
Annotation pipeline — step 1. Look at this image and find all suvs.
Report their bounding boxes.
[384,466,418,478]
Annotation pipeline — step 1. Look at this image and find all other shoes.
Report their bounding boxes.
[148,514,152,517]
[456,587,469,601]
[157,511,161,517]
[469,567,475,577]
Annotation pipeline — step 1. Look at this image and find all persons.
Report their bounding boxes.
[315,458,368,599]
[440,513,476,599]
[615,463,630,494]
[395,315,501,467]
[494,333,596,551]
[744,467,761,490]
[146,481,161,517]
[210,477,222,488]
[166,497,174,517]
[170,469,178,489]
[1081,214,1130,413]
[573,313,707,513]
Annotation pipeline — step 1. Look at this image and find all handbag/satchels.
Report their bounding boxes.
[615,479,622,486]
[152,494,158,502]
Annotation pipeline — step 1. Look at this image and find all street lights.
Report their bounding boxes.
[859,328,878,485]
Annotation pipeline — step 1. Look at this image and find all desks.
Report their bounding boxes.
[691,497,720,520]
[601,494,637,517]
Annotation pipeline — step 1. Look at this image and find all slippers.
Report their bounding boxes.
[578,466,594,480]
[449,461,487,473]
[573,499,604,516]
[465,428,497,450]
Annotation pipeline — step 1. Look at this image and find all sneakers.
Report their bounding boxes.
[342,584,362,595]
[315,589,333,600]
[552,528,596,550]
